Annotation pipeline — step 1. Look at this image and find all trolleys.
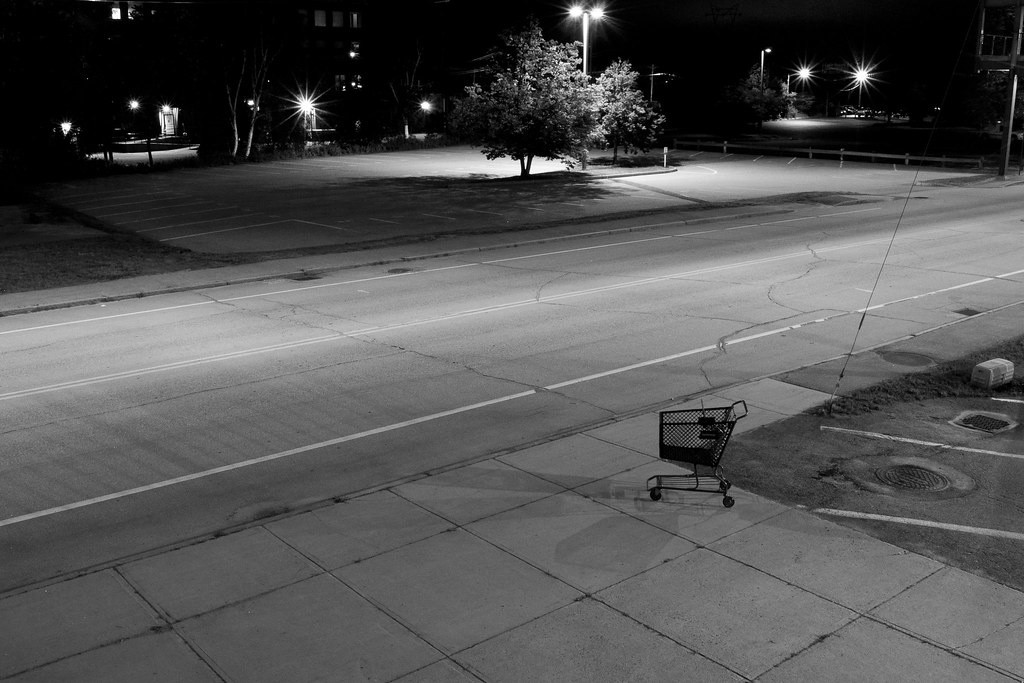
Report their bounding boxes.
[647,400,749,508]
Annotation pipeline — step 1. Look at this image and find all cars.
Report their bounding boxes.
[1011,129,1024,142]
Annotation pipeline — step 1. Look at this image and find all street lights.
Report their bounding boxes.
[569,5,605,88]
[787,69,809,96]
[858,69,867,107]
[761,48,771,95]
